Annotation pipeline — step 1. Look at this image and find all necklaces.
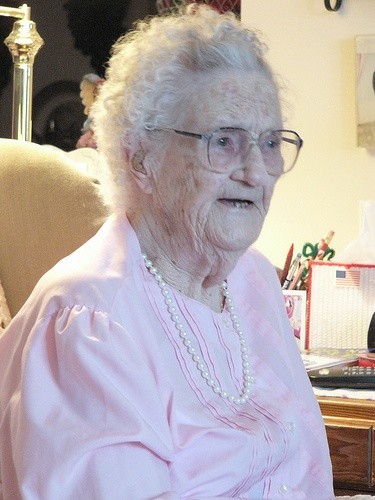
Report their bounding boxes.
[143,253,253,405]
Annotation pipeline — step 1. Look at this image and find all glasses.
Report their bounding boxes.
[156,127,304,177]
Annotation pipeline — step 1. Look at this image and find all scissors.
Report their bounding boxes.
[301,243,335,281]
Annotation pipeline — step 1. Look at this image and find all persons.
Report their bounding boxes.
[0,6,333,498]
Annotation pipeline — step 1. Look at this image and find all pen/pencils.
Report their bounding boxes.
[279,231,334,290]
